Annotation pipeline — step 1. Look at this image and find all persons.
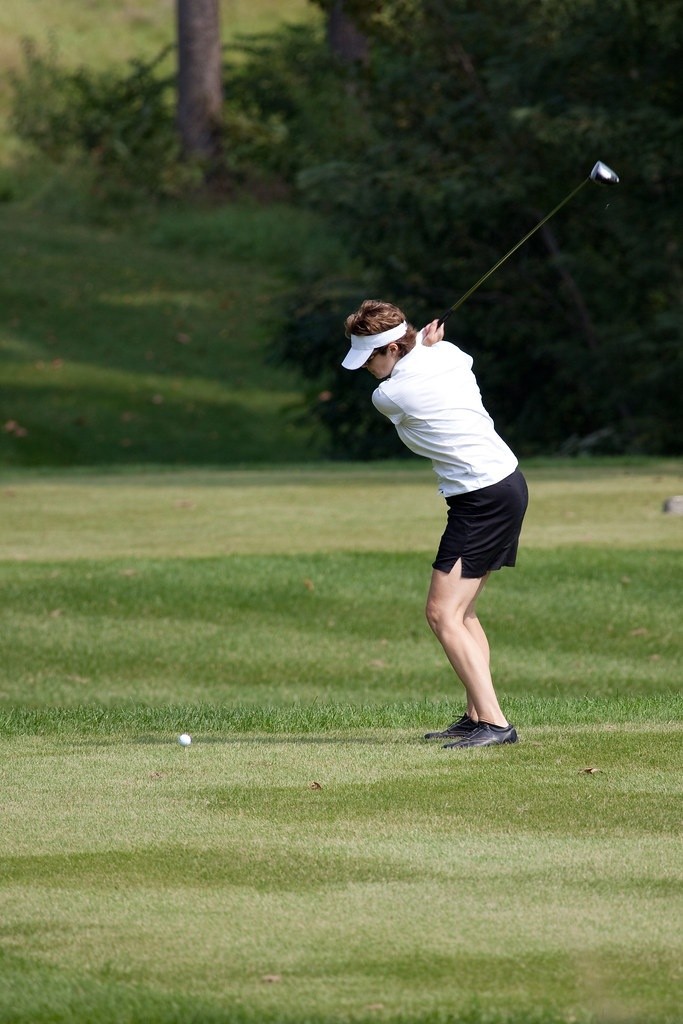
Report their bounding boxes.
[342,301,527,754]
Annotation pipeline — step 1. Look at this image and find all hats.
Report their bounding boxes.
[341,319,408,370]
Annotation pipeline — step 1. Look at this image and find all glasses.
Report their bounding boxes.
[364,350,381,365]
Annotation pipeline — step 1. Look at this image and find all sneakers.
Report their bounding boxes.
[444,719,518,750]
[424,712,478,739]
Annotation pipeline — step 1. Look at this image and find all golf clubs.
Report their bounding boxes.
[437,159,620,328]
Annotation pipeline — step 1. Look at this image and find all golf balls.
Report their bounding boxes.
[178,734,191,746]
